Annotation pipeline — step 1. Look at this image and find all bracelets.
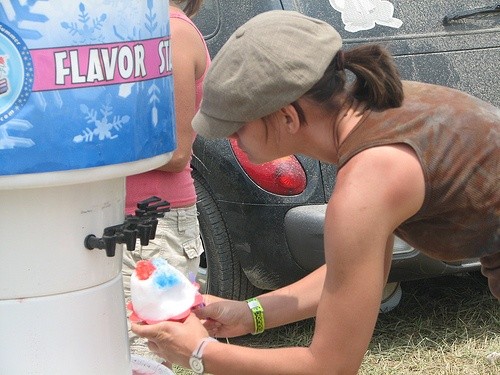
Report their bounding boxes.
[245,297,265,335]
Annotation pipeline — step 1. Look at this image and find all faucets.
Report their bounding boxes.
[84,194,171,257]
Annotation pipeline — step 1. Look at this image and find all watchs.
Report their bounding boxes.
[189,335,216,374]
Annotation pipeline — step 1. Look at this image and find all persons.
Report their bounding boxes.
[121,0,212,375]
[129,9,500,375]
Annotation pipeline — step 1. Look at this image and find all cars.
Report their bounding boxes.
[175,0,500,345]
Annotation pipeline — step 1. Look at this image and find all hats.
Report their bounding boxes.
[191,10,343,139]
